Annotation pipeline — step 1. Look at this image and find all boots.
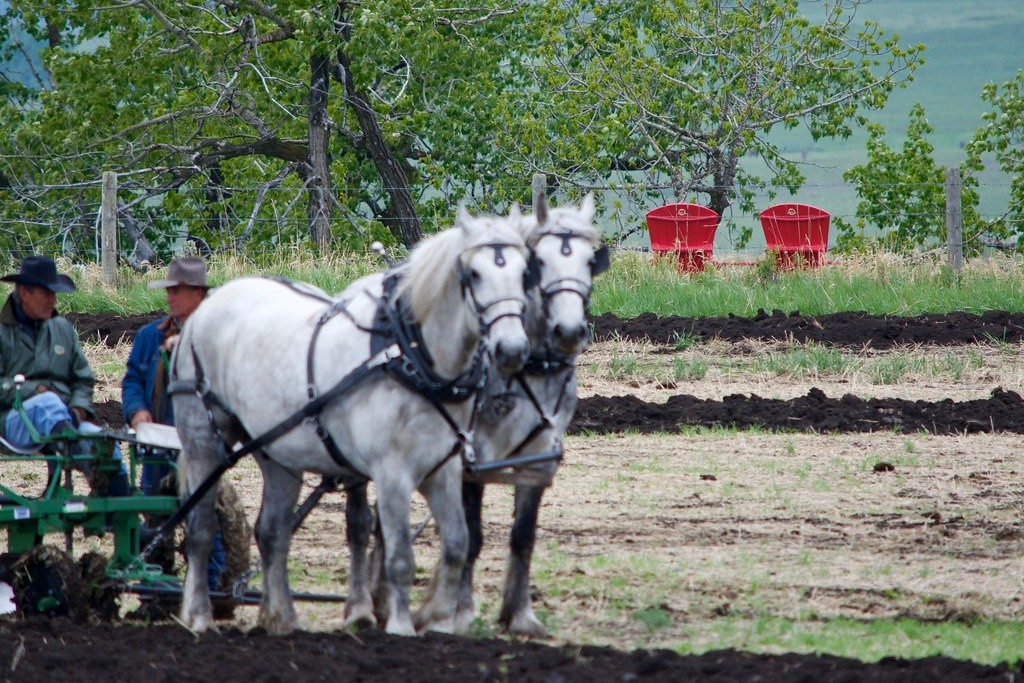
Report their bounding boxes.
[104,472,161,540]
[49,420,121,497]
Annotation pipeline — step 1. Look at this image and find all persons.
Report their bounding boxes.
[121,256,240,618]
[0,255,161,556]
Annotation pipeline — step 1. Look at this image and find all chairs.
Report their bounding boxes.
[646,204,719,272]
[760,203,830,274]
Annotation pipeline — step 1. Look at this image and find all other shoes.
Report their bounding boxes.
[208,593,237,622]
[123,601,181,624]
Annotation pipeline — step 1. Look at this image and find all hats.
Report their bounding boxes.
[0,255,77,294]
[147,255,218,291]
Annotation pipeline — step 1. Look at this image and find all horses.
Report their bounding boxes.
[168,189,602,638]
